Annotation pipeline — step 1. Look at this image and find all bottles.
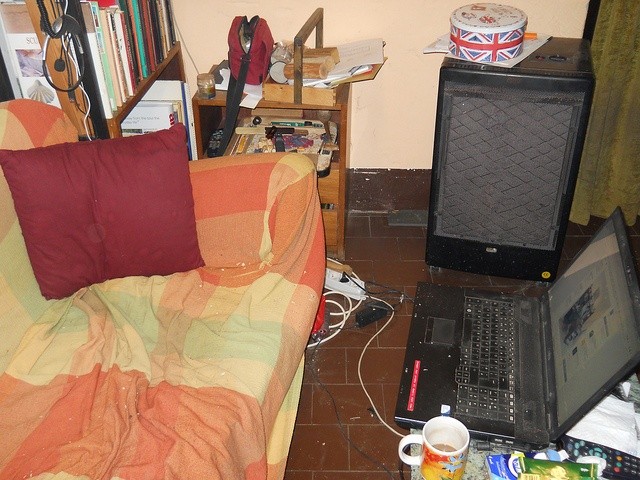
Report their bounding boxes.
[197,73,216,100]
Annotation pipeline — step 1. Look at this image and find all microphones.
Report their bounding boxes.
[53,32,69,72]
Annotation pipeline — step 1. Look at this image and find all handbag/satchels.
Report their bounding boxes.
[228,15,274,85]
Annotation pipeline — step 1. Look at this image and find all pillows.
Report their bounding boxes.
[1,121,207,300]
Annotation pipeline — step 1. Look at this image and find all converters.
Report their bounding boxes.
[355,301,387,328]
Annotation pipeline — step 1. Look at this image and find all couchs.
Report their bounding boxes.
[0,98,329,480]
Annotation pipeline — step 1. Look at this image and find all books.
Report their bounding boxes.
[81,0,176,119]
[287,59,374,87]
[117,46,185,134]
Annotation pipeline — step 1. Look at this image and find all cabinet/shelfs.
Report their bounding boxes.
[189,59,353,263]
[0,0,188,140]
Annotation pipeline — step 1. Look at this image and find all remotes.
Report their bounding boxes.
[207,128,224,157]
[556,433,640,480]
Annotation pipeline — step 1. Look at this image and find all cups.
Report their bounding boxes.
[398,416,471,480]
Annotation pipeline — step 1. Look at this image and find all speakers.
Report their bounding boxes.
[425,37,596,283]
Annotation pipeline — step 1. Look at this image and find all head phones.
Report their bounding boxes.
[42,15,85,92]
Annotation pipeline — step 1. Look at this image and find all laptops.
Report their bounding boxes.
[393,206,640,450]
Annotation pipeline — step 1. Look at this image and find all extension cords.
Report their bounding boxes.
[325,267,366,300]
